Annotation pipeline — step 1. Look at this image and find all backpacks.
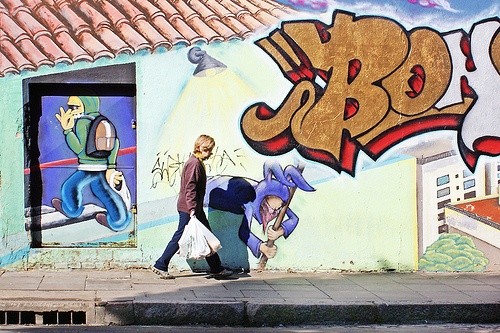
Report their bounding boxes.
[76,115,117,158]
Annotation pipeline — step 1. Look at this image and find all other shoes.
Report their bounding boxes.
[211,269,233,276]
[151,264,175,279]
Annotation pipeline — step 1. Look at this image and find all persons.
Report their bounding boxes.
[148,135,234,279]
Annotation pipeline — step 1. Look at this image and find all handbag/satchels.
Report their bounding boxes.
[177,214,223,260]
